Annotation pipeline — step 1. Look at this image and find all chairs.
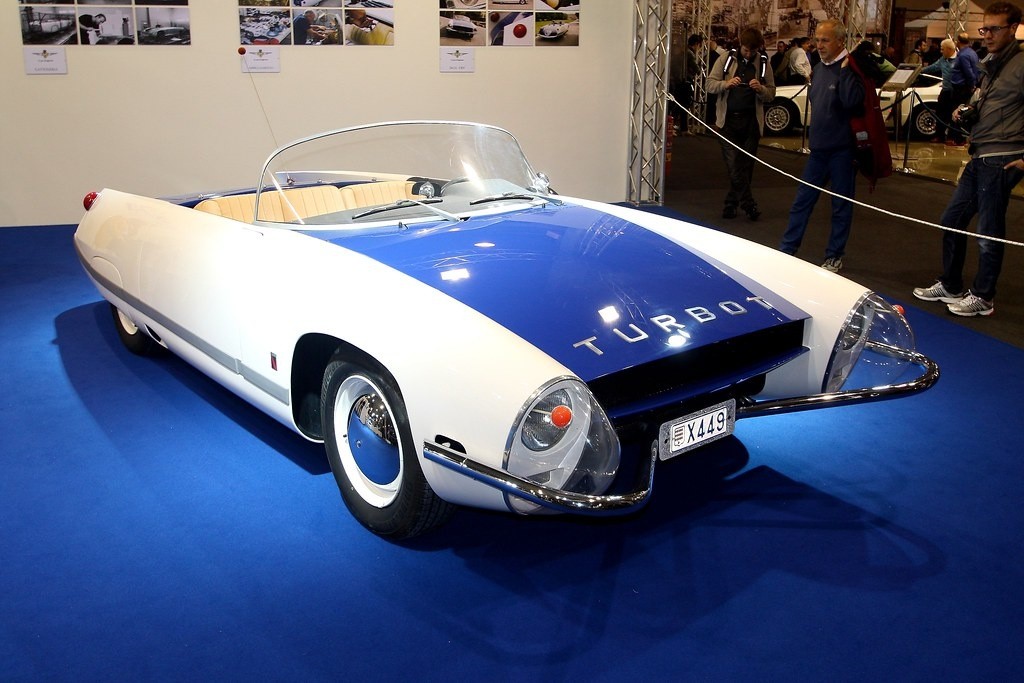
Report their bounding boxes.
[195,181,426,223]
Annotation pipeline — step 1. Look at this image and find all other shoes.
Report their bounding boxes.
[720,206,737,218]
[731,199,768,220]
[946,138,966,146]
[930,137,943,143]
[681,130,696,137]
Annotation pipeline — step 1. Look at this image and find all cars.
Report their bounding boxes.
[764,72,980,138]
[445,14,478,39]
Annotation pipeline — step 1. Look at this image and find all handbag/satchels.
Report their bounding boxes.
[843,54,894,179]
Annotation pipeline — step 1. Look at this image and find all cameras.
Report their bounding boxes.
[957,106,980,129]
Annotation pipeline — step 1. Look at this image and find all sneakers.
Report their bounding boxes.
[912,279,964,303]
[820,256,843,273]
[947,288,994,316]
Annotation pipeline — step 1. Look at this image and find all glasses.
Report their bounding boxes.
[978,25,1010,36]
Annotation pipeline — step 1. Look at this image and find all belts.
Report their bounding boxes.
[730,112,741,119]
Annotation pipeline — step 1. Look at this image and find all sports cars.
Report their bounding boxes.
[72,120,942,544]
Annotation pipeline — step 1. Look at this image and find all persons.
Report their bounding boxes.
[776,20,872,271]
[293,10,327,45]
[920,39,956,142]
[912,3,1024,316]
[78,13,107,44]
[950,33,981,145]
[680,33,932,145]
[705,28,776,221]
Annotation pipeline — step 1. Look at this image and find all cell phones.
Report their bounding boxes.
[737,83,750,88]
